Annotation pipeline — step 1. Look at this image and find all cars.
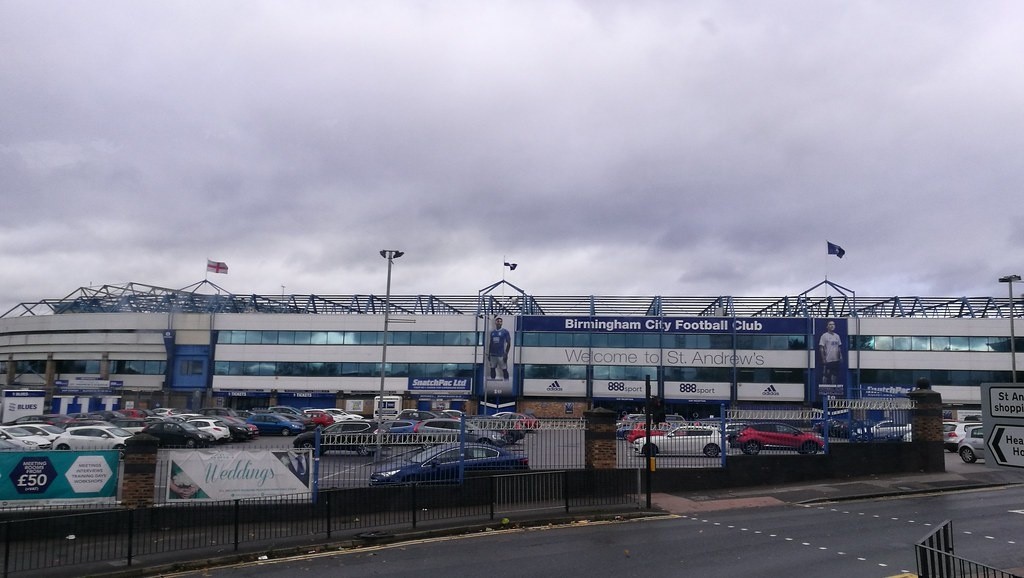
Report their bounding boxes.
[0,396,542,456]
[614,408,984,463]
[371,441,532,486]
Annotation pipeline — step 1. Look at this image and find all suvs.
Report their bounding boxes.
[736,421,824,455]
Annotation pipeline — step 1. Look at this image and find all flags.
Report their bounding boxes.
[827,241,845,259]
[207,259,229,274]
[504,262,517,270]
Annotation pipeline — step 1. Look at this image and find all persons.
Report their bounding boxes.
[488,318,511,382]
[819,321,843,385]
[170,468,200,499]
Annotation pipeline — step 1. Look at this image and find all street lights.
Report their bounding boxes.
[373,249,404,463]
[997,273,1024,382]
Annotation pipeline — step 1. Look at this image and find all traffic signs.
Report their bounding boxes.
[979,382,1024,471]
